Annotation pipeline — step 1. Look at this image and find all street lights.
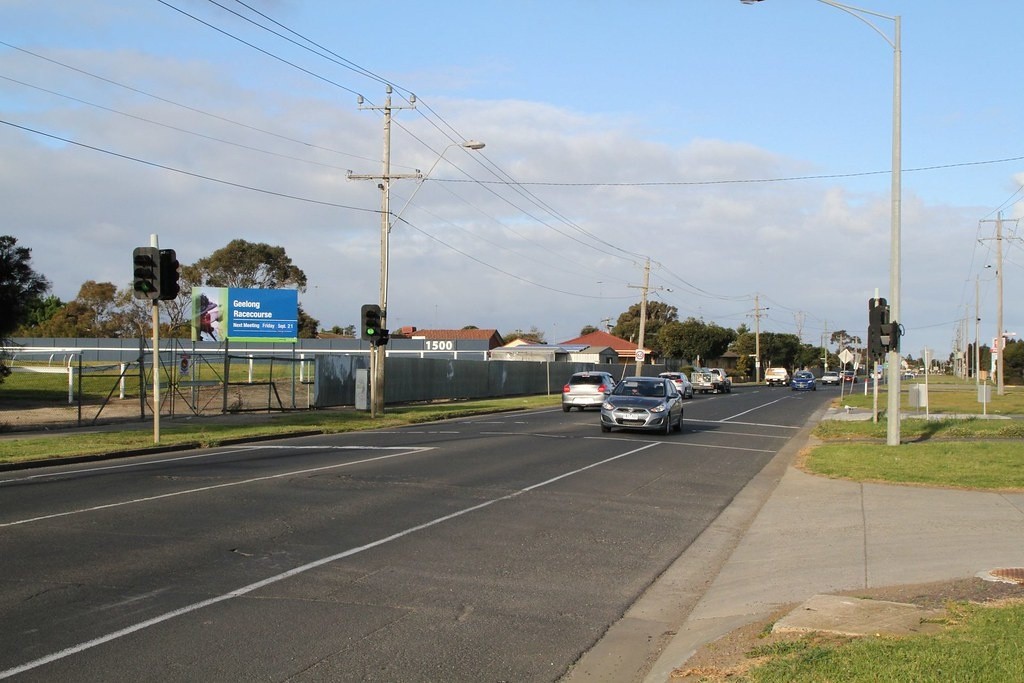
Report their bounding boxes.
[373,142,487,417]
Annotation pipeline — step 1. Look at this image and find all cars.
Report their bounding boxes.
[561,371,627,413]
[841,372,858,383]
[869,365,881,380]
[839,372,849,379]
[790,370,816,391]
[905,369,918,376]
[821,371,840,386]
[653,371,694,399]
[599,376,684,435]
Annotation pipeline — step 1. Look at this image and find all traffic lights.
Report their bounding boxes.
[375,329,389,346]
[880,321,897,349]
[161,248,180,301]
[133,246,161,301]
[360,304,382,341]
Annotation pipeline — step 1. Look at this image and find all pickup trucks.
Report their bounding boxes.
[765,368,790,387]
[690,367,732,395]
